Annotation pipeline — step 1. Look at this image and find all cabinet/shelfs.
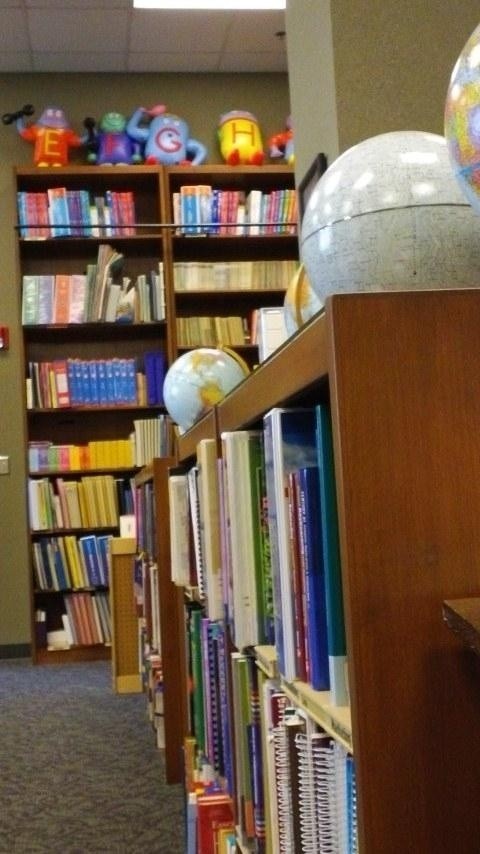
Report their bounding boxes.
[17,160,301,668]
[131,457,183,784]
[175,288,480,852]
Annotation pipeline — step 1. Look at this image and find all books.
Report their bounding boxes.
[16,183,300,747]
[183,604,358,853]
[294,401,349,709]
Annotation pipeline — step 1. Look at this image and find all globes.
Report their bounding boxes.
[442,23,480,221]
[284,265,323,337]
[298,130,480,306]
[163,345,251,431]
